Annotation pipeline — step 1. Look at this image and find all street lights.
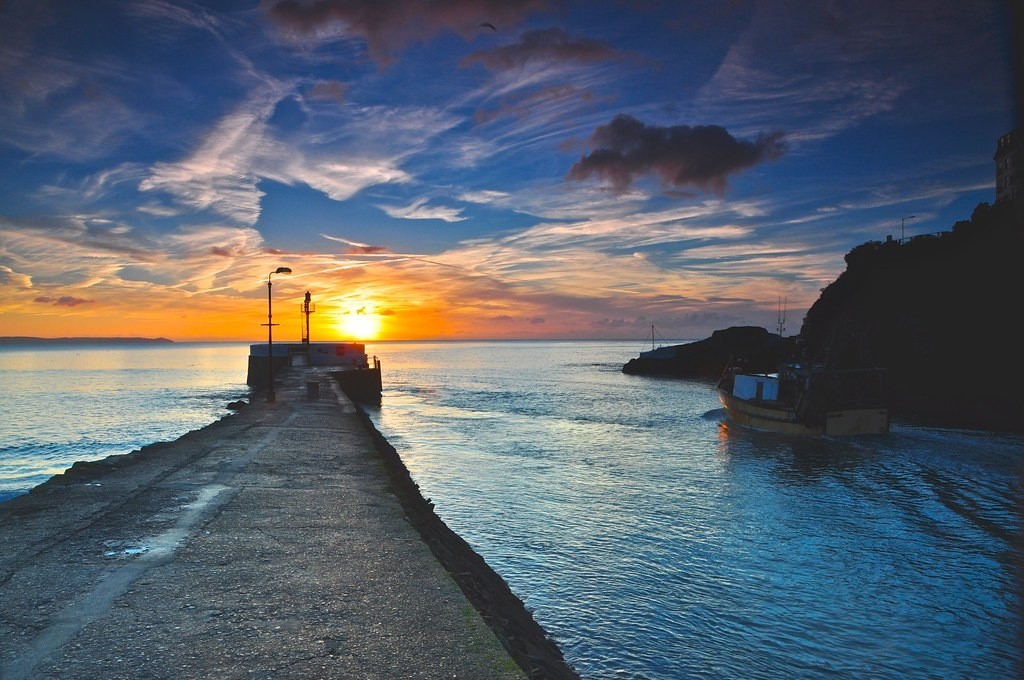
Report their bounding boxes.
[267,266,293,402]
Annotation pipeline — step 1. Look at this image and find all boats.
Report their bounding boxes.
[714,341,893,438]
